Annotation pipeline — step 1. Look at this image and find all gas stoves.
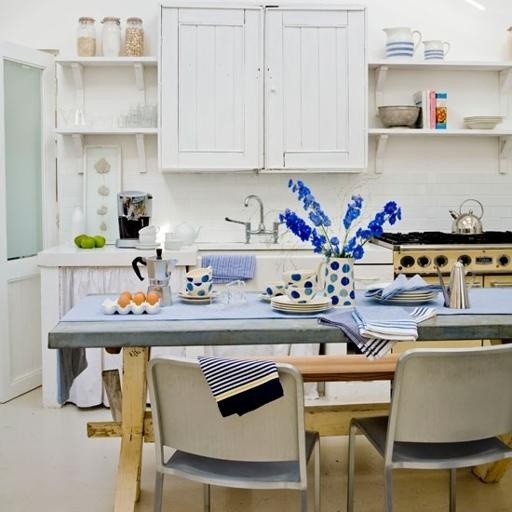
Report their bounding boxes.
[374,230,511,268]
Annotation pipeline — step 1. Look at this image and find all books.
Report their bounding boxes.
[412,89,448,130]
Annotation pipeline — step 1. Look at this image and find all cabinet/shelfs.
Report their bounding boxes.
[322,261,397,401]
[52,55,158,172]
[366,57,512,175]
[152,3,372,179]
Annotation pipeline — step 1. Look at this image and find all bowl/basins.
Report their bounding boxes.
[164,239,182,251]
[378,106,420,128]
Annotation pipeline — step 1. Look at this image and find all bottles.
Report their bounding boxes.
[434,93,448,128]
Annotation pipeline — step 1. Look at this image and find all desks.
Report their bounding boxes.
[42,286,512,512]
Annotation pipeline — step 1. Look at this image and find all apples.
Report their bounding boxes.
[75,235,106,249]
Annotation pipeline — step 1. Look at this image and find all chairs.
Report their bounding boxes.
[142,354,328,512]
[342,342,512,512]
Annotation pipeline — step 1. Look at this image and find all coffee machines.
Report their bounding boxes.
[116,191,153,248]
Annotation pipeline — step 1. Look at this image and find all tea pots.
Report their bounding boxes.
[449,197,484,234]
[131,250,179,307]
[432,262,474,309]
[422,40,450,61]
[383,26,422,64]
[175,221,203,247]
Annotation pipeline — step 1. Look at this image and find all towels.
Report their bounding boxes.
[309,305,436,362]
[380,271,442,298]
[351,305,418,344]
[363,272,408,297]
[201,254,257,285]
[195,354,283,420]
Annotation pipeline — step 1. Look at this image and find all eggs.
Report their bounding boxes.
[117,292,160,308]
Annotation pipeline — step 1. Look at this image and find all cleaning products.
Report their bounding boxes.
[70,205,84,244]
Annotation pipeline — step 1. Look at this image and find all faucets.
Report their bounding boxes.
[244,193,265,234]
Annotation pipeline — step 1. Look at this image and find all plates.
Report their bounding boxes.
[272,295,332,314]
[138,243,161,250]
[462,115,503,130]
[257,293,271,301]
[372,289,438,304]
[178,291,218,303]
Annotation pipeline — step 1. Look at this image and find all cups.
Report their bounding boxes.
[110,102,158,128]
[185,267,213,297]
[226,280,246,305]
[137,225,161,244]
[165,232,173,241]
[267,271,325,303]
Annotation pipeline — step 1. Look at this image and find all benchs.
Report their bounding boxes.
[227,353,401,406]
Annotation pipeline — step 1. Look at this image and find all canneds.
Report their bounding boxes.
[101,17,121,57]
[125,17,144,57]
[77,16,96,57]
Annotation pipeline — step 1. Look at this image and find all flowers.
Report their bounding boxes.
[271,176,407,264]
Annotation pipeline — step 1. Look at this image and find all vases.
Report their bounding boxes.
[323,258,357,305]
